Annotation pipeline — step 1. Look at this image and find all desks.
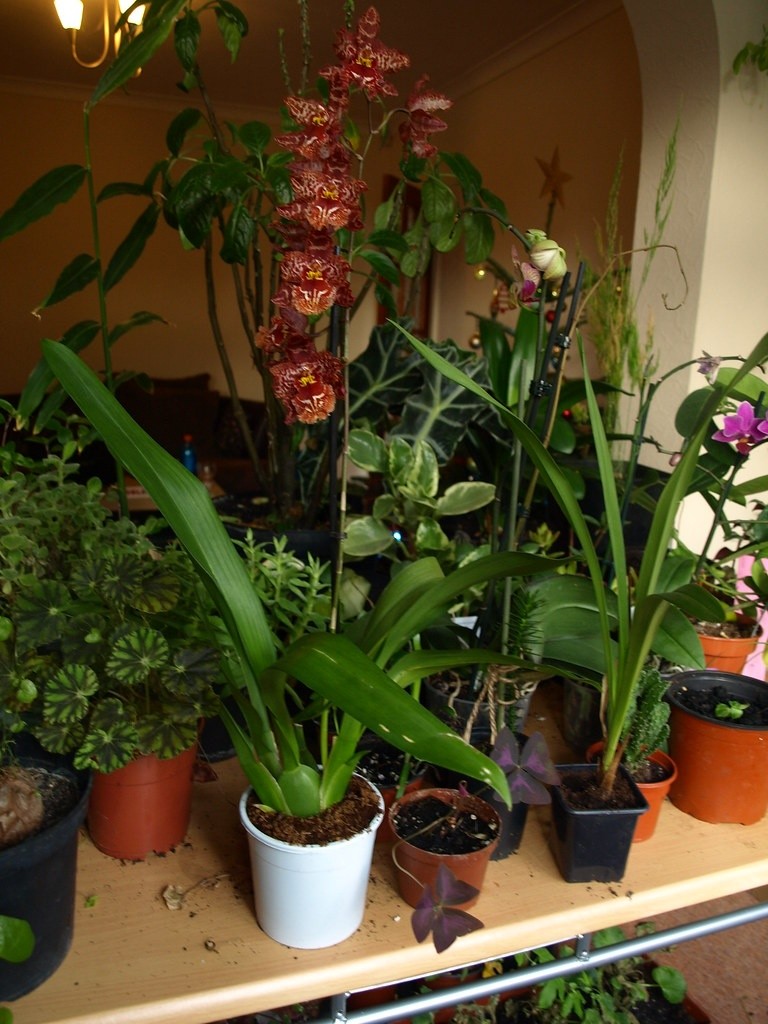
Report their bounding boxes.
[0,680,767,1024]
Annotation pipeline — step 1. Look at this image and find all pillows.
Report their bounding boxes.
[114,384,221,457]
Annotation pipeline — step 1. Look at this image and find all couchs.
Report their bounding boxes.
[0,371,271,499]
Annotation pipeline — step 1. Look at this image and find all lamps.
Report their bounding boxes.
[52,0,148,78]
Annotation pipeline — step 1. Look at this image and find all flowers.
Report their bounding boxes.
[265,5,453,733]
[666,349,768,619]
[458,208,569,325]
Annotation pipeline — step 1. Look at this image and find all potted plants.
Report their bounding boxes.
[0,0,768,1024]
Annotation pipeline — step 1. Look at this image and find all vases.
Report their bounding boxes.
[684,610,764,674]
